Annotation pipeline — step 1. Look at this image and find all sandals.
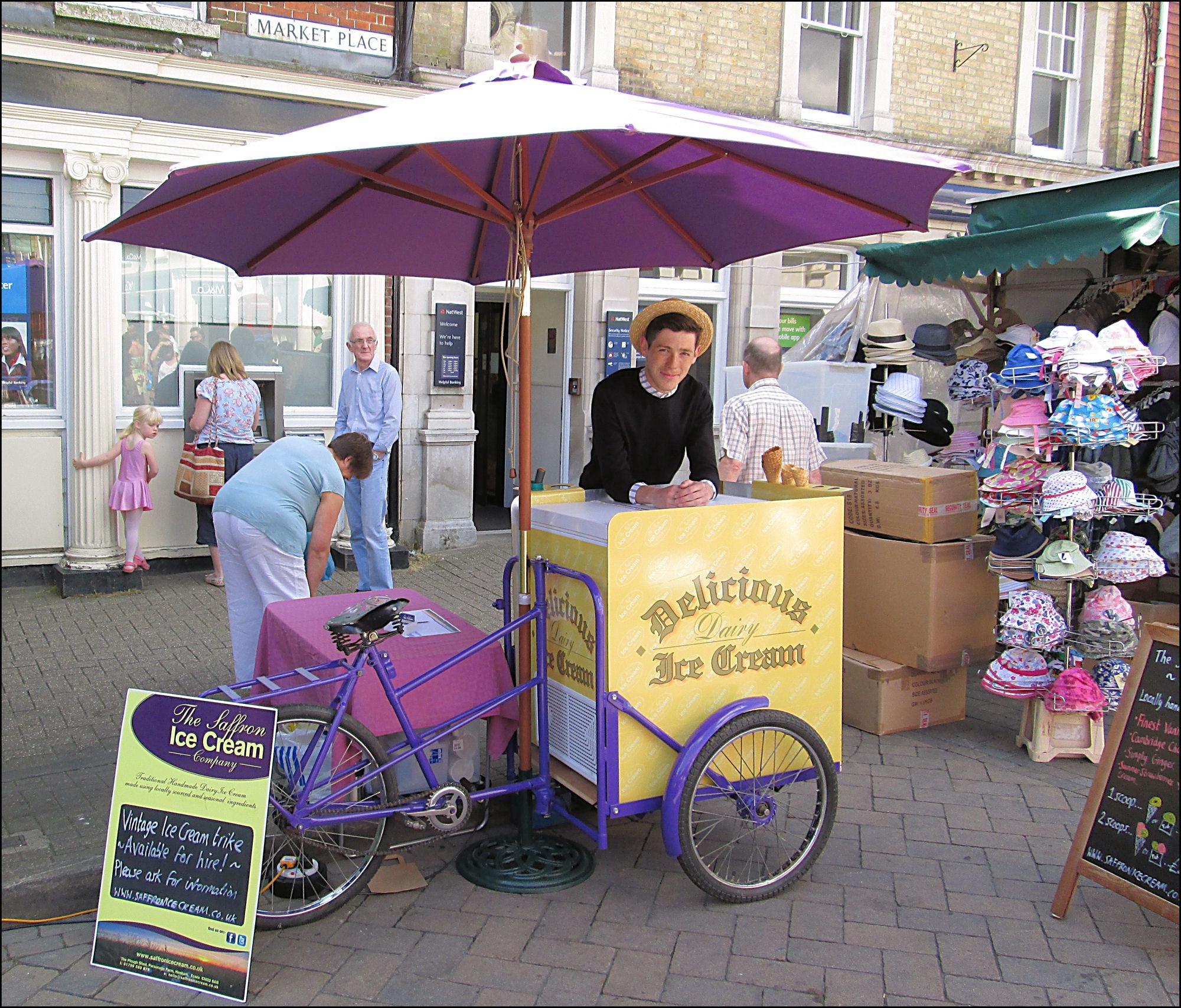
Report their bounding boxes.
[134,554,150,570]
[122,562,137,573]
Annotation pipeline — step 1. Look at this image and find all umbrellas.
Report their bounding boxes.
[80,43,975,896]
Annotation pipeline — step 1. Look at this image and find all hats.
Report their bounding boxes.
[630,298,714,358]
[861,279,1180,713]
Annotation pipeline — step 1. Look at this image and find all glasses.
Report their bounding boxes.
[350,339,376,346]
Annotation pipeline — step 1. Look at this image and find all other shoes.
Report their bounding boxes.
[205,576,224,587]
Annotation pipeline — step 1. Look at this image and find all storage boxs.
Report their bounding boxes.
[819,455,982,545]
[841,639,976,735]
[838,525,1003,677]
[355,730,451,800]
[721,360,876,462]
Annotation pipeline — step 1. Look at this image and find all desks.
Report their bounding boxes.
[252,590,524,855]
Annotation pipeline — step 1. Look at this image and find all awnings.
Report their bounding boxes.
[854,167,1180,323]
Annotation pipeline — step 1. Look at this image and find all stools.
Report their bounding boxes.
[1016,694,1106,769]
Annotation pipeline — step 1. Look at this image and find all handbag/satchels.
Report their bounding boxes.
[172,377,227,506]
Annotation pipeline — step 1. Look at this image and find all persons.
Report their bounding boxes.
[120,323,323,407]
[71,405,164,573]
[330,323,402,592]
[190,341,262,585]
[577,298,722,511]
[0,326,28,383]
[716,335,829,484]
[210,431,374,691]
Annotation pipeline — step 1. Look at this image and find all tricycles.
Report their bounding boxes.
[195,471,855,933]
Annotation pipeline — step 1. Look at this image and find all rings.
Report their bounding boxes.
[372,452,379,461]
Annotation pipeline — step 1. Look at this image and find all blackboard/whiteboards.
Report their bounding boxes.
[1069,622,1181,924]
